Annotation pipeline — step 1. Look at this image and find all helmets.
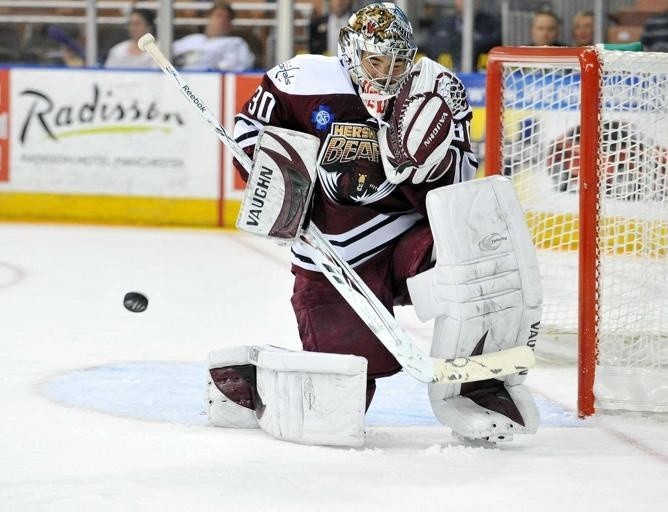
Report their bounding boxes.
[337,3,418,104]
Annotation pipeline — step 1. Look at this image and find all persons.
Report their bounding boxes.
[528,9,593,47]
[100,7,161,69]
[310,1,503,74]
[200,3,548,450]
[172,3,257,72]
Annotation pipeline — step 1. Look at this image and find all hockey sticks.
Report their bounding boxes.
[137,33,537,384]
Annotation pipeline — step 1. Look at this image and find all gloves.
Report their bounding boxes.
[380,92,455,186]
